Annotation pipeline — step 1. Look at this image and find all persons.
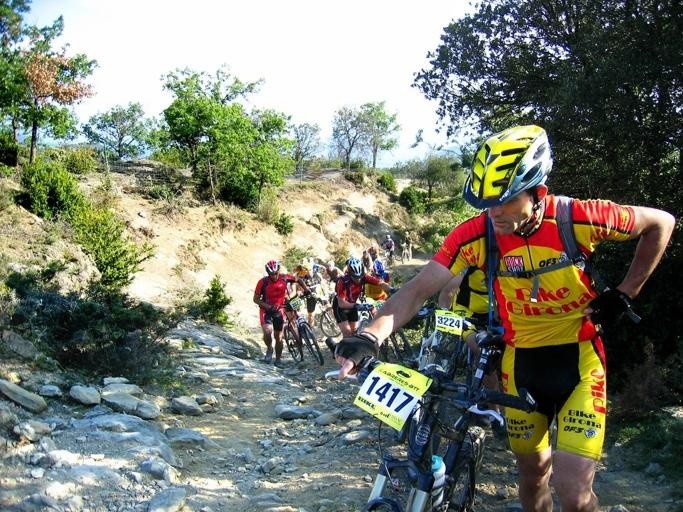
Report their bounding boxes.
[380,235,394,258]
[401,231,412,260]
[331,124,675,512]
[252,245,400,369]
[437,265,506,437]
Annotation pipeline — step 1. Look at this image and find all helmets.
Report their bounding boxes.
[265,231,409,279]
[462,125,553,209]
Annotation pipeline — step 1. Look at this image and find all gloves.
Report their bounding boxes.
[589,289,633,336]
[334,332,380,369]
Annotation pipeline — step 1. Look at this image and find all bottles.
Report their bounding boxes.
[430,453,447,508]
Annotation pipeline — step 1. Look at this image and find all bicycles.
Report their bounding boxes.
[323,327,536,511]
[354,298,419,370]
[392,300,488,465]
[298,288,345,336]
[270,289,324,368]
[361,242,412,285]
[324,279,335,303]
